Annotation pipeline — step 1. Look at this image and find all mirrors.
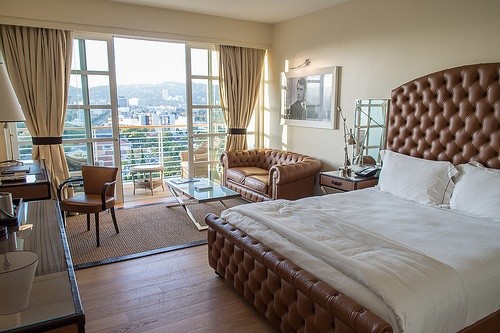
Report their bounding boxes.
[352,99,390,178]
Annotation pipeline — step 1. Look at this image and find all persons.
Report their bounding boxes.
[289,77,320,120]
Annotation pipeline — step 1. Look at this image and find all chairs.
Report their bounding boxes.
[66,153,99,171]
[57,165,118,247]
[179,139,222,179]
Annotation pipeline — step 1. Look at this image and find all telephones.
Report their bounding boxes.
[354,165,381,178]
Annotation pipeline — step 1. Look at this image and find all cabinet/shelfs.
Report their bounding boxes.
[0,200,86,333]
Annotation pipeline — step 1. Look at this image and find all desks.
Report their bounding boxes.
[129,165,165,196]
[0,159,52,203]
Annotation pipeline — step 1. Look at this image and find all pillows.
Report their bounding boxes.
[448,161,500,222]
[376,150,459,209]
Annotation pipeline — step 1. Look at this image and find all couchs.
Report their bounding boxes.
[220,149,322,203]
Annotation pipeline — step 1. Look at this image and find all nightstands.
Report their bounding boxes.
[319,171,379,196]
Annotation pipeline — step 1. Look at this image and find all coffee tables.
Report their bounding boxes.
[165,177,241,231]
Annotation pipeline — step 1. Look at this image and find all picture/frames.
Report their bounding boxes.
[280,66,339,130]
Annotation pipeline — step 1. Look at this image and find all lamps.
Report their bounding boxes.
[0,62,26,237]
[0,236,39,315]
[336,106,356,177]
[354,106,386,173]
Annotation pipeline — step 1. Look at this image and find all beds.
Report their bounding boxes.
[205,62,500,333]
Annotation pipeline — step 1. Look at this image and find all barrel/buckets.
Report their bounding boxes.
[0,192,14,218]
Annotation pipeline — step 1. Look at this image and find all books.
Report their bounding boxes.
[192,182,213,191]
[170,176,200,184]
